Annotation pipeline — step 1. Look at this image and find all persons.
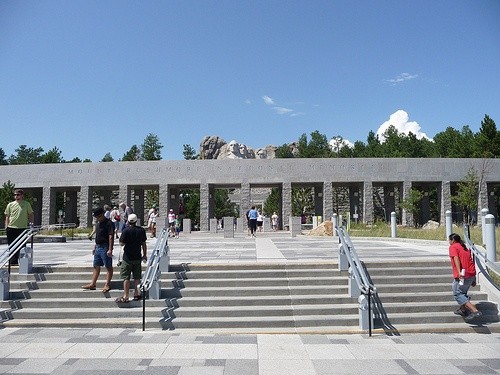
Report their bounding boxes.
[116,214,147,303]
[168,209,181,238]
[272,211,280,231]
[227,141,267,159]
[104,202,133,238]
[302,214,306,224]
[148,205,159,237]
[449,233,482,321]
[4,189,34,265]
[88,230,95,241]
[82,208,115,293]
[247,206,263,238]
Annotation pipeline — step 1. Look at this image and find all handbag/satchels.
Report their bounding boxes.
[114,215,121,221]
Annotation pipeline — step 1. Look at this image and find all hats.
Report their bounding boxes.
[169,209,174,212]
[273,212,277,214]
[92,207,105,216]
[128,214,137,223]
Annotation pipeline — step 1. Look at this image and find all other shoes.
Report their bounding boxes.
[133,295,141,300]
[82,283,96,290]
[102,285,111,292]
[454,308,481,323]
[115,297,129,303]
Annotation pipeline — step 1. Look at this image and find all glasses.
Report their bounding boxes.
[13,194,20,196]
